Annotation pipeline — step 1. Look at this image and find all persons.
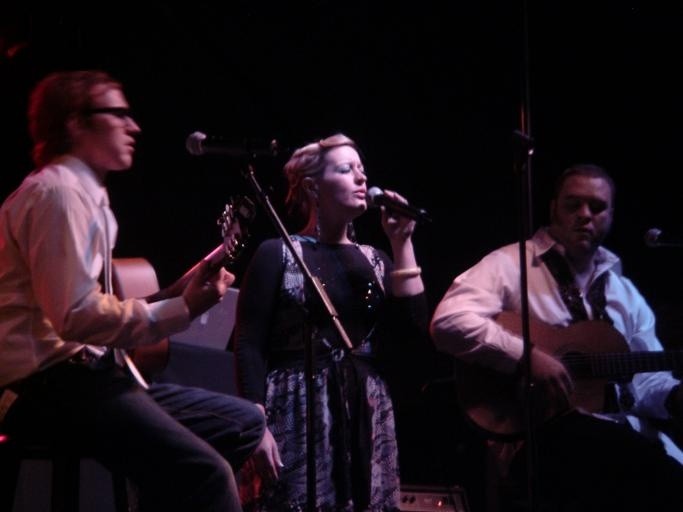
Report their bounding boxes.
[429,164,682,511]
[235,132,428,511]
[0,70,267,509]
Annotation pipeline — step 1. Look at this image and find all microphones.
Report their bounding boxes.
[643,226,683,248]
[365,186,435,226]
[185,131,278,157]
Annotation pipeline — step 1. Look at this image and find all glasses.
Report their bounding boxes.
[81,103,132,118]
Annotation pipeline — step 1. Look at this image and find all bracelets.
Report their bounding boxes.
[392,266,422,277]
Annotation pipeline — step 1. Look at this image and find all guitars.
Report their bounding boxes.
[111,194,278,379]
[453,311,682,439]
